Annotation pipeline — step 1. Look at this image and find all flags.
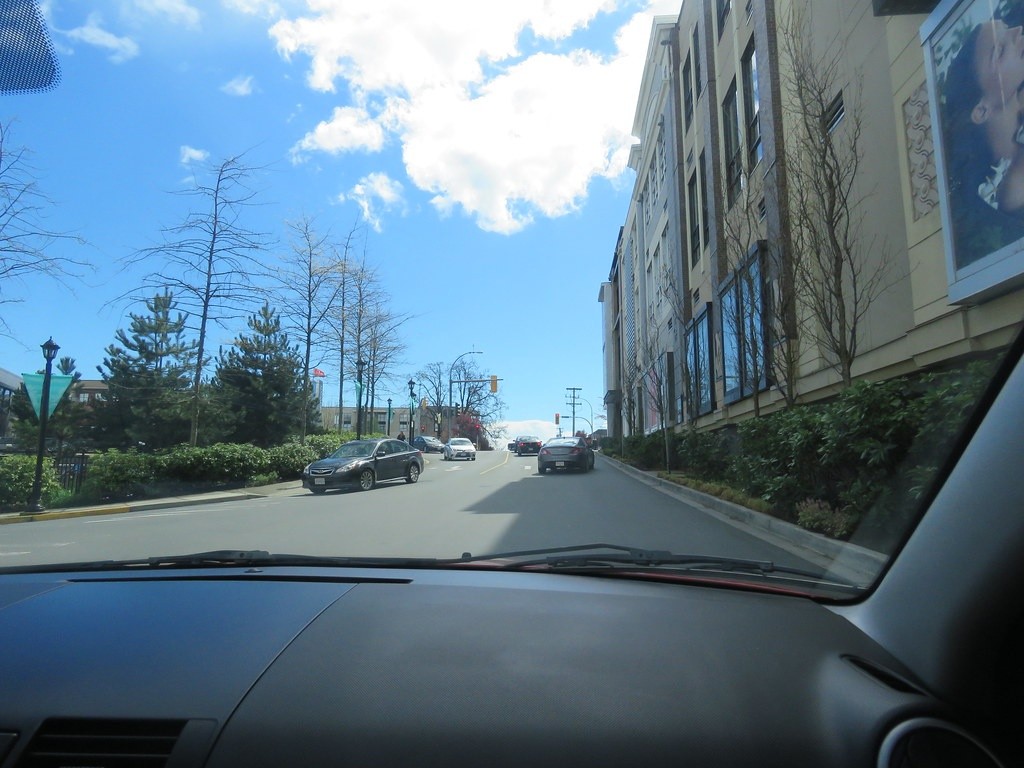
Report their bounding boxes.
[314,368,324,377]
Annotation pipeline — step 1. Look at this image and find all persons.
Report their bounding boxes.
[397,431,405,441]
[946,20,1024,215]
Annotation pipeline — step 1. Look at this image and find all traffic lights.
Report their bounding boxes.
[475,424,481,431]
[555,413,560,425]
[451,409,455,417]
[456,402,461,417]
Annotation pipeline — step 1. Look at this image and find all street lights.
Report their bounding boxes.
[409,391,417,447]
[387,398,394,437]
[18,335,61,517]
[354,357,368,441]
[407,377,416,447]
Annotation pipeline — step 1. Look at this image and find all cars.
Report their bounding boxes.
[414,435,447,455]
[513,436,526,453]
[444,437,478,461]
[301,439,424,493]
[538,436,596,471]
[518,436,543,457]
